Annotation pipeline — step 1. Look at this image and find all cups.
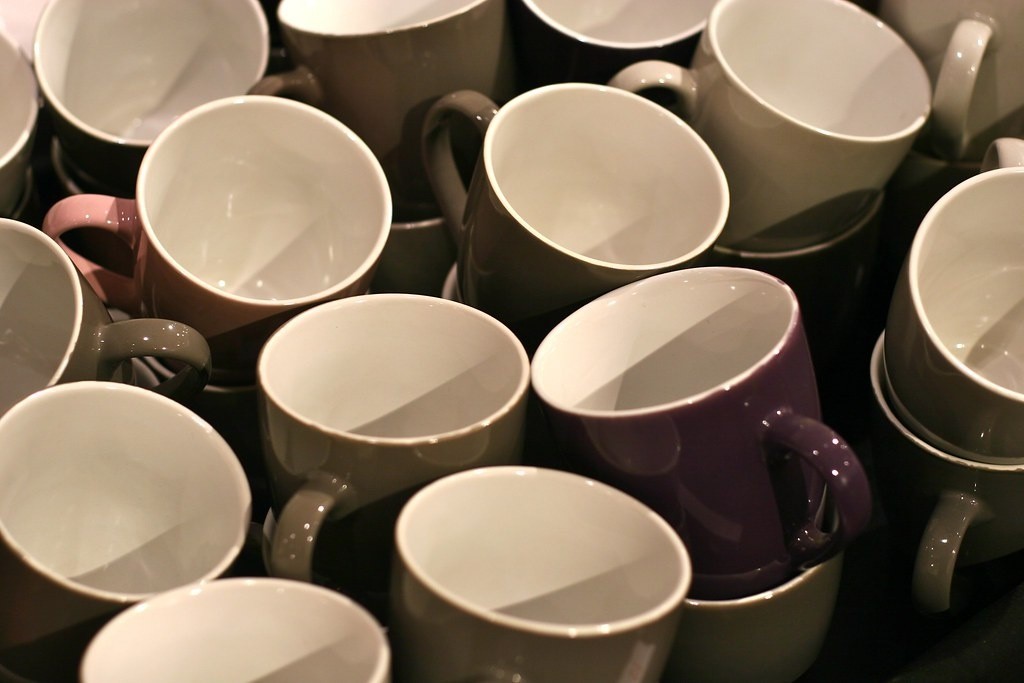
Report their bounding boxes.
[0,0,1024,683]
